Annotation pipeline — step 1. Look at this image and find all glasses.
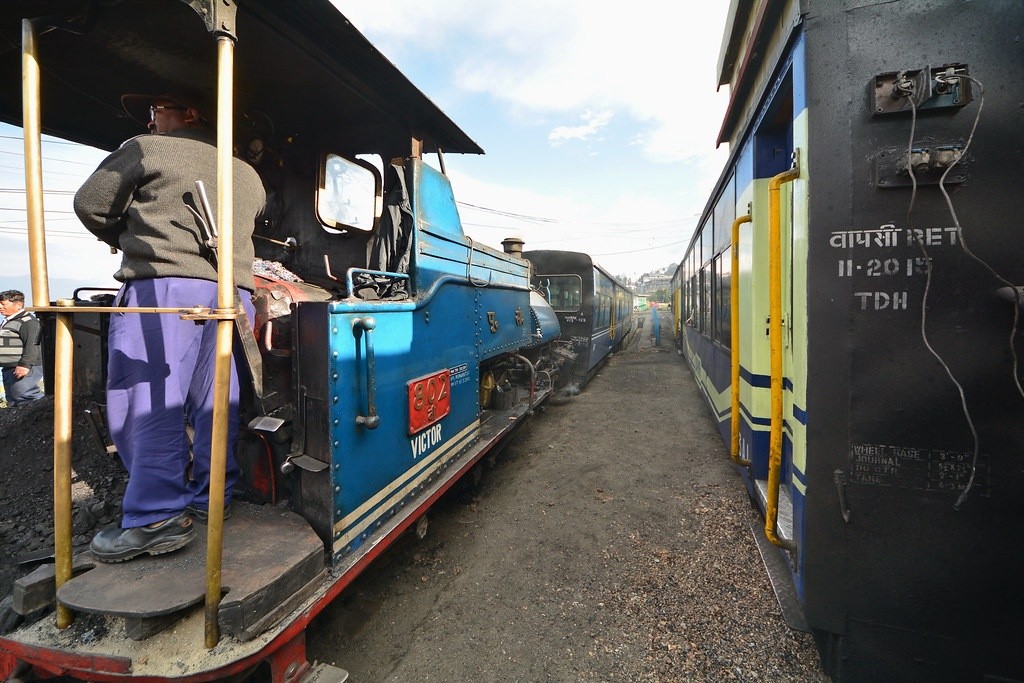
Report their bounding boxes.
[148,106,187,123]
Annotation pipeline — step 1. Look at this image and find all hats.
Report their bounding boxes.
[119,63,209,133]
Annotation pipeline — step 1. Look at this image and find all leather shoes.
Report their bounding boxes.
[185,505,232,526]
[89,510,198,564]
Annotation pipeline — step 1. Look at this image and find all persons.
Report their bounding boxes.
[0,290,46,409]
[73,88,266,563]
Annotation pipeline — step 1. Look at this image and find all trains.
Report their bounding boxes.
[0,0,633,682]
[668,0,1024,682]
